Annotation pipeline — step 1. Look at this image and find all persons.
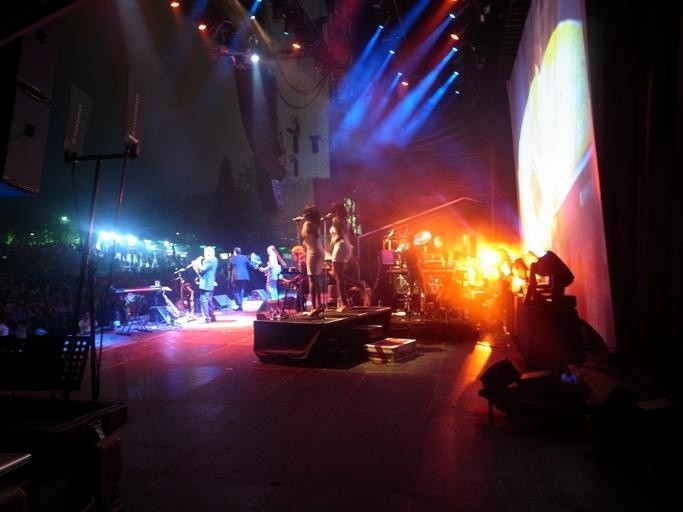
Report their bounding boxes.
[324,201,353,312]
[281,245,309,313]
[396,231,429,299]
[294,203,326,317]
[258,244,286,300]
[76,311,98,333]
[191,247,219,323]
[227,246,259,311]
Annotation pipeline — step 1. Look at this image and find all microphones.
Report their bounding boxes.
[291,217,304,222]
[320,213,332,222]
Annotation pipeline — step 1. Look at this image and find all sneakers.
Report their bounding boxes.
[203,306,244,324]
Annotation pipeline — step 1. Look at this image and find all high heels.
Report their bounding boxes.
[306,304,351,317]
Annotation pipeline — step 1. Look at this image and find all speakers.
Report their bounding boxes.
[0,83,50,195]
[0,0,57,101]
[212,295,232,309]
[149,307,169,323]
[251,289,269,300]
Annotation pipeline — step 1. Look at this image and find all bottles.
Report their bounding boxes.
[377,300,382,308]
